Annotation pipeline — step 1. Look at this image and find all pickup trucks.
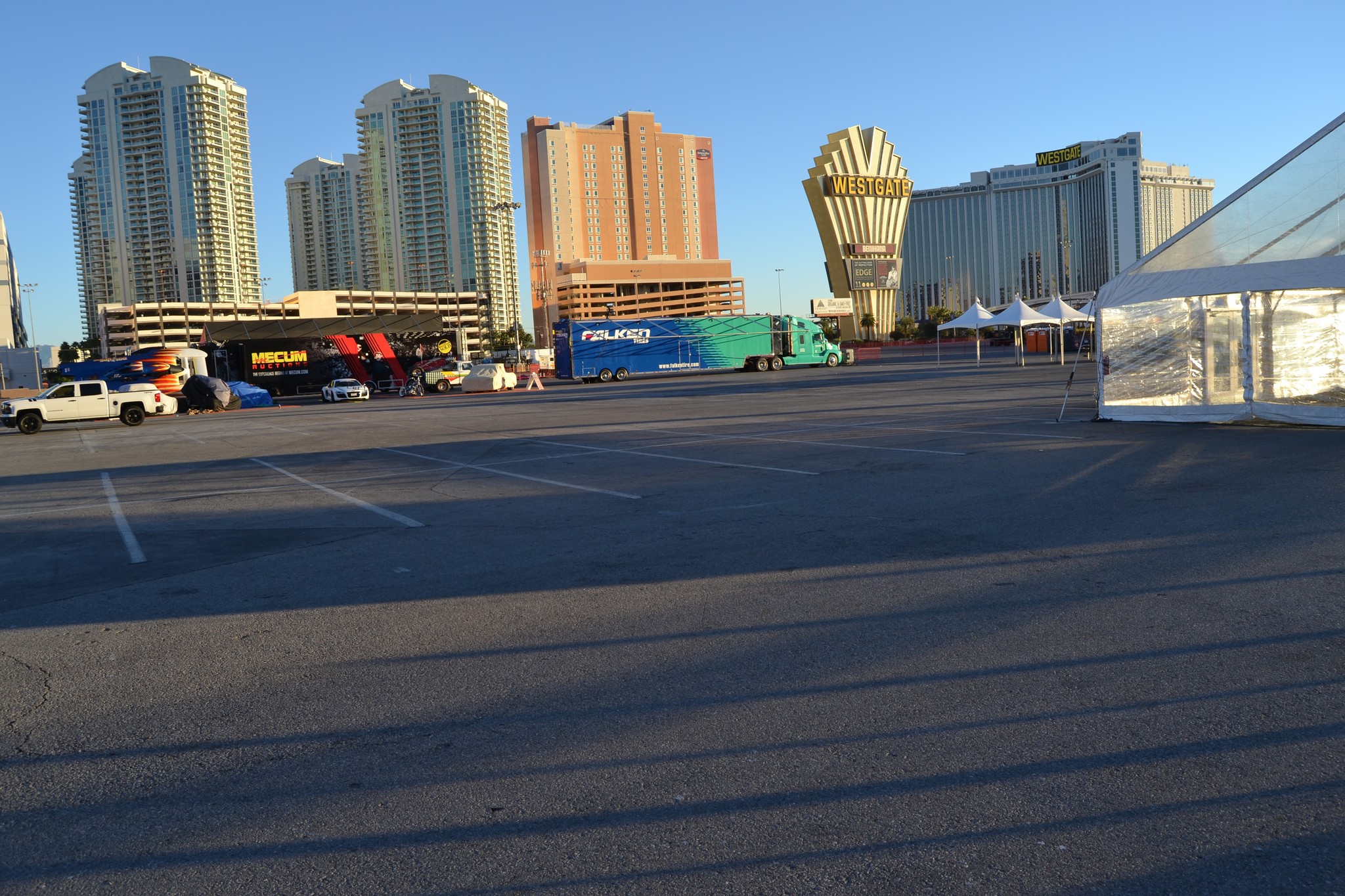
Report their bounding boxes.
[0,380,164,434]
[422,360,474,393]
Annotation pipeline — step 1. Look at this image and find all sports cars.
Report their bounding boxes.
[321,379,369,403]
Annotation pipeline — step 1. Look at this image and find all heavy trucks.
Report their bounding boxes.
[552,312,843,384]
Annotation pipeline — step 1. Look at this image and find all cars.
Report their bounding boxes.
[461,363,517,393]
[925,337,944,344]
[408,357,447,379]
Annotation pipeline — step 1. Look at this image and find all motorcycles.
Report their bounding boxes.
[399,376,424,397]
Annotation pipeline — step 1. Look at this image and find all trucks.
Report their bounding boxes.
[899,339,914,346]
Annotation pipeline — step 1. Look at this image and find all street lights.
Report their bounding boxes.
[253,277,271,320]
[1060,240,1072,327]
[945,256,956,338]
[239,264,247,302]
[775,269,785,318]
[630,270,643,319]
[17,284,41,392]
[418,264,425,292]
[493,202,521,363]
[347,262,355,291]
[158,270,165,303]
[439,273,454,331]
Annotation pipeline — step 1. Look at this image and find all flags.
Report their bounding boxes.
[420,344,423,353]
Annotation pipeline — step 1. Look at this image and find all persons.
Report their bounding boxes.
[307,342,439,388]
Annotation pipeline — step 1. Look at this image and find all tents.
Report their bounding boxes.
[1097,115,1345,426]
[937,291,1095,369]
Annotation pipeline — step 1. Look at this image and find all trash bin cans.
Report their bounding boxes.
[1026,328,1037,352]
[1063,326,1074,351]
[1047,327,1056,352]
[1036,327,1048,352]
[1053,327,1065,351]
